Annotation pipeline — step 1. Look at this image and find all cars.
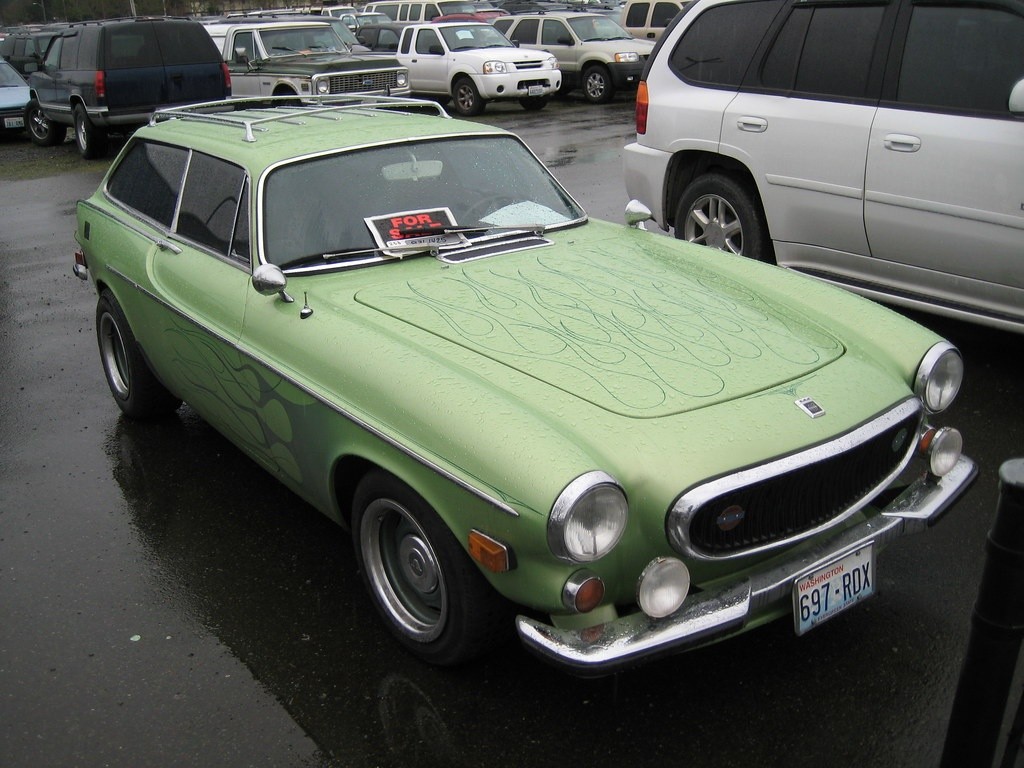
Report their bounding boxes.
[72,94,980,671]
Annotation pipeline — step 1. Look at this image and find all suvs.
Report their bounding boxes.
[0,0,693,162]
[622,0,1023,330]
